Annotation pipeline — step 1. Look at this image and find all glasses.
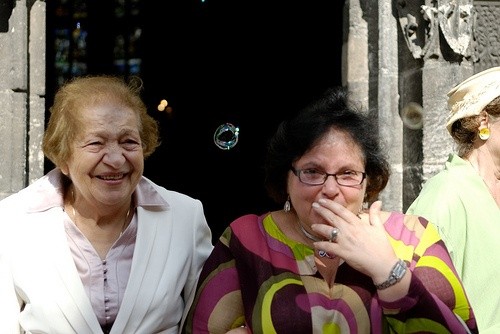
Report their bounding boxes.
[290,166,366,187]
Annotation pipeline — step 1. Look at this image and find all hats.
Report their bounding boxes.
[446,67,500,135]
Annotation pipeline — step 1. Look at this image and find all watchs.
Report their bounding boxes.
[375,258,408,290]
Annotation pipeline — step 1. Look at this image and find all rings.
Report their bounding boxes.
[331,228,339,243]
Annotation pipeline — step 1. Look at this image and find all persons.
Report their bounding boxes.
[184,89,480,334]
[405,67,499,334]
[0,75,214,334]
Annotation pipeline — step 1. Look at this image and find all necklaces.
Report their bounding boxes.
[465,156,500,209]
[71,183,131,240]
[295,211,339,259]
